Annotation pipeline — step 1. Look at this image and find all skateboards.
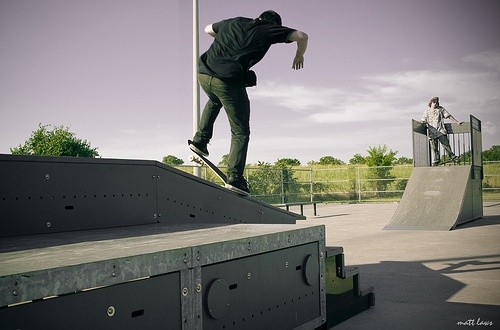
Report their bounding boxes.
[188,139,250,197]
[433,156,459,166]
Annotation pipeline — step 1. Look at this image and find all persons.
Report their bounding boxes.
[421,95,465,165]
[190,9,309,197]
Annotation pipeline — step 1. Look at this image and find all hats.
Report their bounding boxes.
[428,97,439,108]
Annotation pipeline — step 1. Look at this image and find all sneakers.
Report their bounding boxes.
[190,141,209,156]
[225,173,251,197]
[450,154,456,159]
[432,160,440,164]
